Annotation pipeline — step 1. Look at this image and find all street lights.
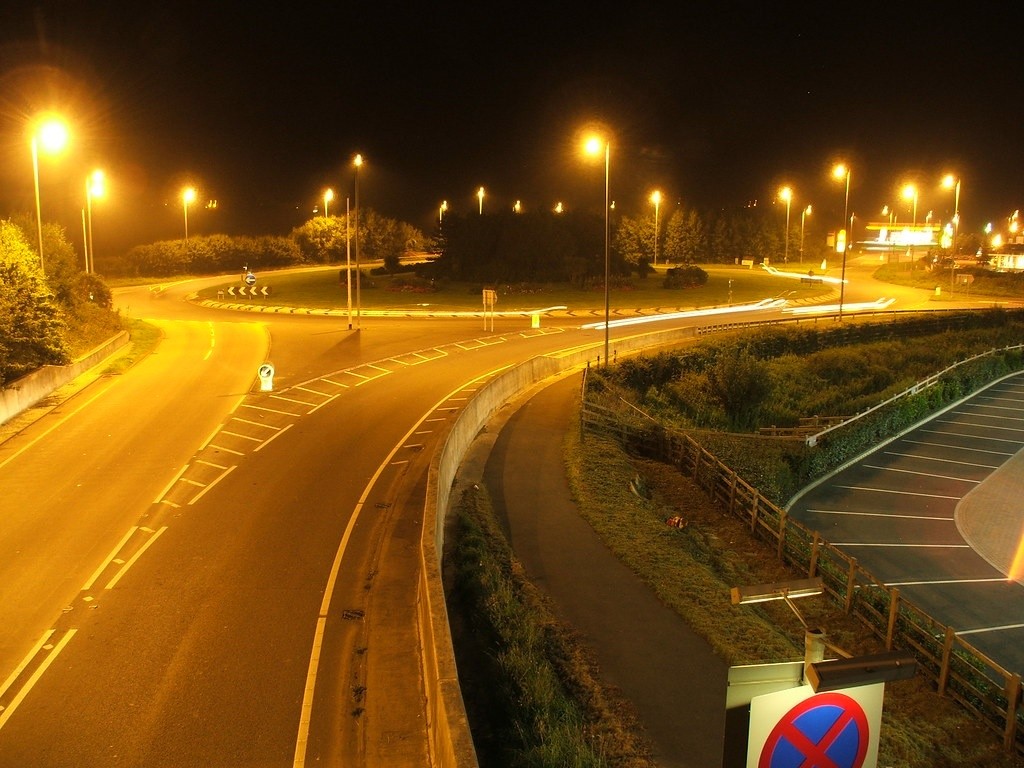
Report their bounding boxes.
[479,187,484,214]
[653,191,660,265]
[942,176,961,297]
[882,188,918,287]
[440,201,447,232]
[31,125,63,279]
[799,205,814,266]
[324,190,333,218]
[86,173,102,275]
[780,188,791,266]
[833,166,851,323]
[355,153,364,331]
[584,140,611,370]
[183,192,192,239]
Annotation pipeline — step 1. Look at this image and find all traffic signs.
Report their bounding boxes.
[227,286,274,295]
